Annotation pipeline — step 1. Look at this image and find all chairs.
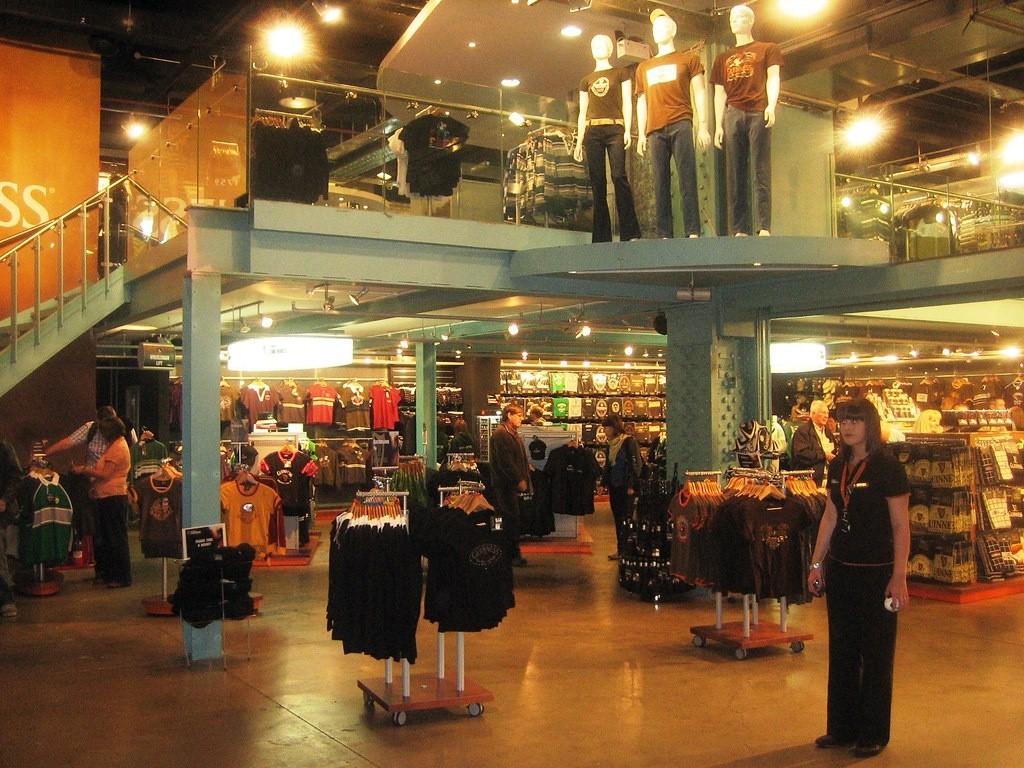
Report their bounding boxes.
[250,108,316,132]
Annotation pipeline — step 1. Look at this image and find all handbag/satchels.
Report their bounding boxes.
[170,543,257,630]
[625,436,652,491]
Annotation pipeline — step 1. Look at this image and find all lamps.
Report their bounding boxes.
[321,283,336,311]
[257,303,273,329]
[236,307,251,333]
[349,283,369,306]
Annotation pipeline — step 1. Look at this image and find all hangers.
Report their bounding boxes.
[220,376,392,391]
[397,452,478,477]
[135,427,297,491]
[950,374,973,386]
[315,438,360,450]
[683,466,821,506]
[920,375,944,385]
[520,120,576,137]
[893,375,912,387]
[425,108,446,118]
[349,478,423,529]
[527,432,579,473]
[866,376,887,388]
[838,377,865,389]
[980,374,1024,383]
[173,378,184,385]
[443,483,496,516]
[22,462,57,480]
[894,199,943,212]
[836,178,891,208]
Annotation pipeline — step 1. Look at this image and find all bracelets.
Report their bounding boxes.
[809,562,822,570]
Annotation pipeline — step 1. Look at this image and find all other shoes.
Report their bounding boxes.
[93,578,111,584]
[512,559,528,567]
[854,744,886,758]
[106,581,131,588]
[815,735,855,747]
[608,551,618,559]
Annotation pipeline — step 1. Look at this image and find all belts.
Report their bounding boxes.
[586,118,624,127]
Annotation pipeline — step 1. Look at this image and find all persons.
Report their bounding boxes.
[791,398,840,492]
[573,6,780,246]
[951,397,1015,432]
[596,415,643,562]
[911,410,943,434]
[0,405,132,618]
[490,403,546,568]
[806,398,909,757]
[404,412,479,473]
